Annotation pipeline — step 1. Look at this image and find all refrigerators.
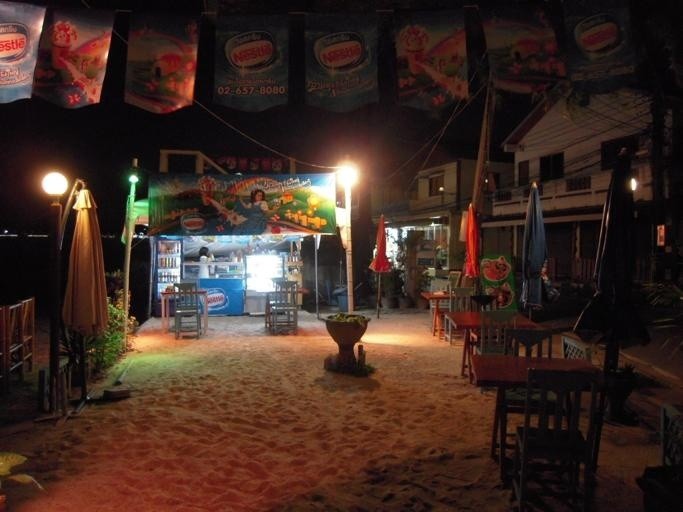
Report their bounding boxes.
[154,236,183,318]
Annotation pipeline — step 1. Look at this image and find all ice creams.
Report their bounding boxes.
[400,24,429,74]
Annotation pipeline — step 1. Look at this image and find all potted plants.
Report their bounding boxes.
[327,314,367,373]
[381,270,413,309]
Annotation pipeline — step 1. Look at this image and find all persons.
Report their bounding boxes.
[198,247,214,278]
[232,190,280,235]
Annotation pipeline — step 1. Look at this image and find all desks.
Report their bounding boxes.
[264,292,302,331]
[161,290,209,335]
[420,291,605,487]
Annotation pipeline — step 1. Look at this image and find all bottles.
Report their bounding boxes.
[288,249,298,292]
[158,241,181,302]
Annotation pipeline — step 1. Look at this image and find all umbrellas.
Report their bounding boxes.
[62,190,110,397]
[369,214,392,319]
[520,181,545,320]
[574,148,651,467]
[465,203,480,279]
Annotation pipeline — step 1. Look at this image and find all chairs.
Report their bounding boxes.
[0,297,36,397]
[514,366,602,511]
[173,282,203,340]
[272,277,298,335]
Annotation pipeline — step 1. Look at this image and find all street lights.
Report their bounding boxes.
[42,171,68,411]
[336,158,361,315]
[119,159,140,352]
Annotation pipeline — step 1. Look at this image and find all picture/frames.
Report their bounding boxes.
[447,269,463,288]
